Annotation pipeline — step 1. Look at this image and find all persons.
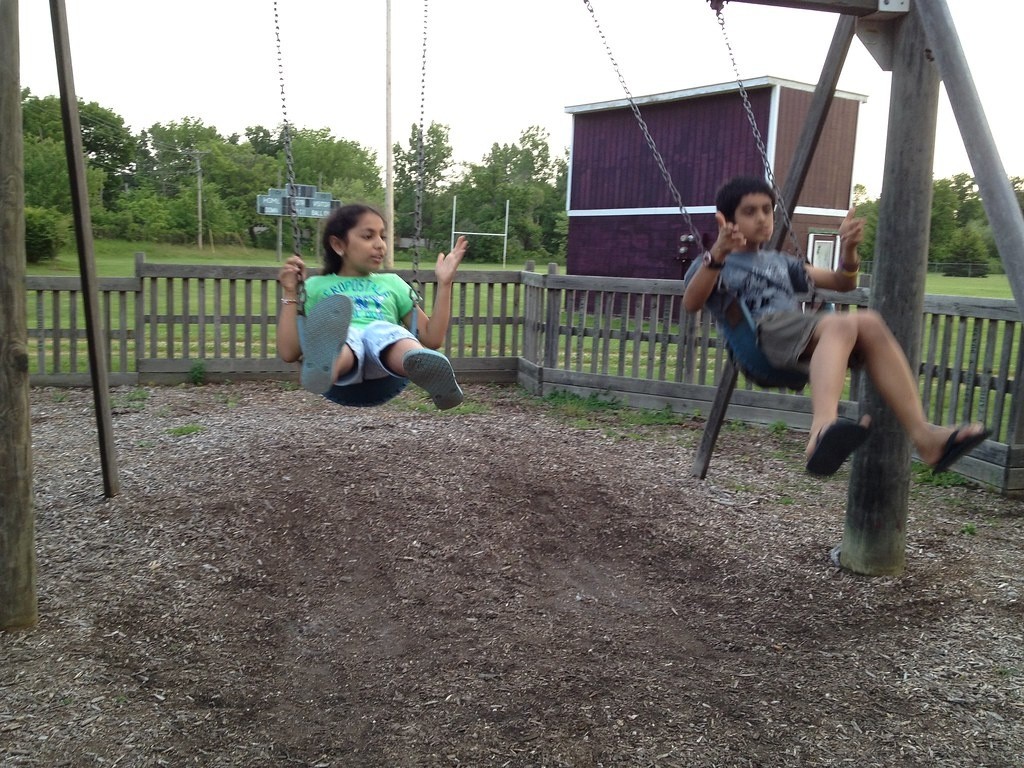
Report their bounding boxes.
[276,204,470,412]
[681,176,995,479]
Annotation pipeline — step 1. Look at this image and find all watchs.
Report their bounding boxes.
[703,250,726,270]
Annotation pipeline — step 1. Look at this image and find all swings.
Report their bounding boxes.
[272,1,430,406]
[584,1,835,387]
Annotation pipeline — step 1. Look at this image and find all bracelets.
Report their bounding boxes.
[280,298,298,306]
[838,256,860,276]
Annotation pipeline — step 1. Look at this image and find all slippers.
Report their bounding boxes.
[932,426,993,475]
[806,418,869,477]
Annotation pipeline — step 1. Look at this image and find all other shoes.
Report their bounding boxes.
[401,349,464,410]
[301,294,353,394]
